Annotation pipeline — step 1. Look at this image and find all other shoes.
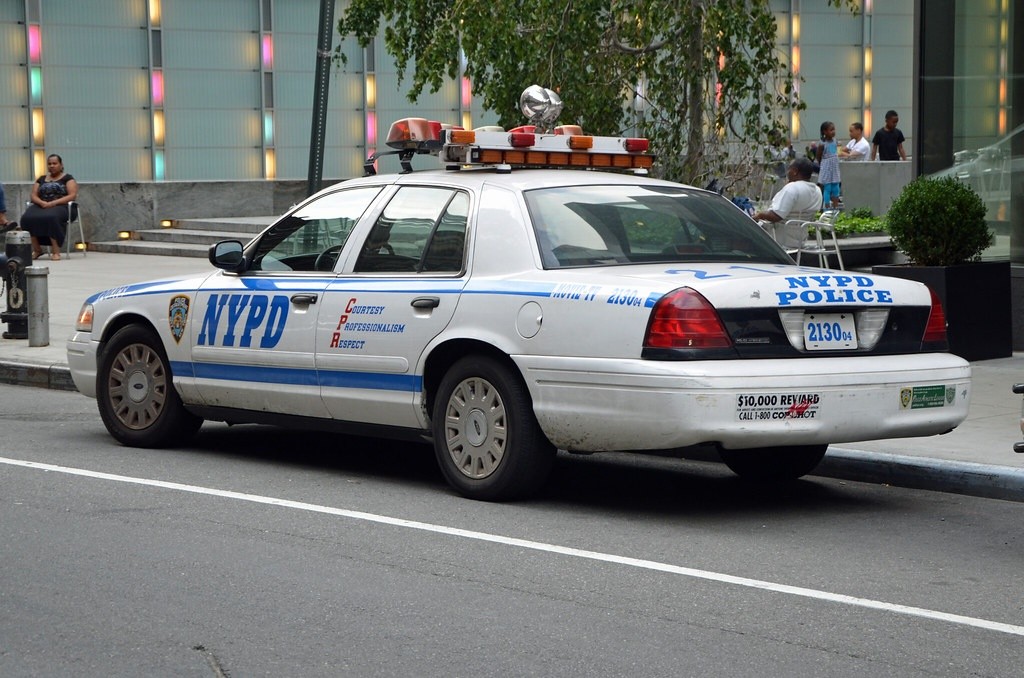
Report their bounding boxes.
[0,220,18,234]
[31,248,44,260]
[51,252,60,260]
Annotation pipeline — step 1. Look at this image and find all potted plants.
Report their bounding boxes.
[872,174,1014,362]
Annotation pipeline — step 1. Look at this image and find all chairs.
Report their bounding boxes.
[421,230,465,272]
[758,210,816,269]
[783,210,844,270]
[758,162,788,203]
[26,201,85,261]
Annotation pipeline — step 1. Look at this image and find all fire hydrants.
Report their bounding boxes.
[0,230,34,339]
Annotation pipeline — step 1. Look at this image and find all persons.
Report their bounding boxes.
[871,110,906,161]
[20,154,76,260]
[0,182,18,233]
[817,121,839,208]
[840,122,870,161]
[752,157,822,249]
[766,129,791,160]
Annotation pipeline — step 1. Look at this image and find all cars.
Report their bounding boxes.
[61,85,974,500]
[928,121,1024,223]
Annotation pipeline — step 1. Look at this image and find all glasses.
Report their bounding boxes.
[786,164,800,173]
